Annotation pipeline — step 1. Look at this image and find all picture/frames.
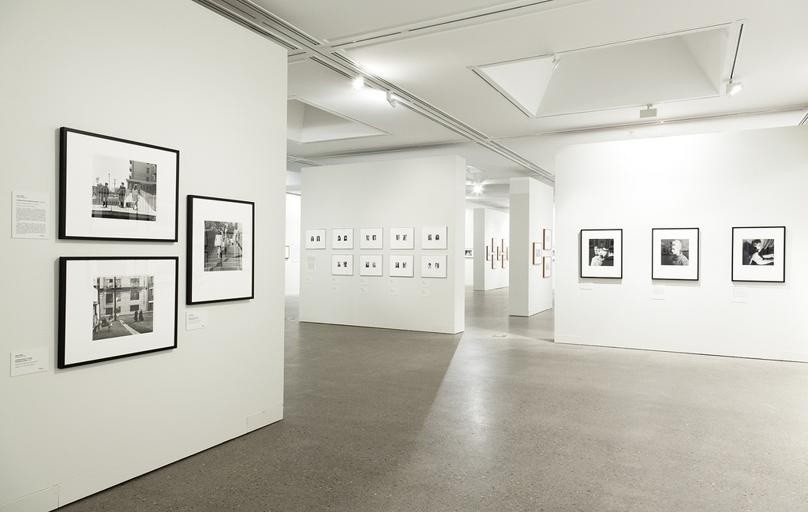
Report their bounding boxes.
[652,227,699,280]
[186,195,254,305]
[732,225,786,283]
[57,256,178,369]
[580,229,623,279]
[58,126,179,243]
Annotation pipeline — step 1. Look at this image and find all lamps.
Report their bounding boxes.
[727,78,742,97]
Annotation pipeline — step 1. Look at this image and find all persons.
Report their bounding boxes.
[134,309,138,321]
[213,230,224,261]
[93,300,100,335]
[748,240,772,264]
[669,240,689,266]
[232,225,239,261]
[116,182,125,208]
[139,309,143,321]
[590,245,614,266]
[131,183,139,210]
[100,182,109,207]
